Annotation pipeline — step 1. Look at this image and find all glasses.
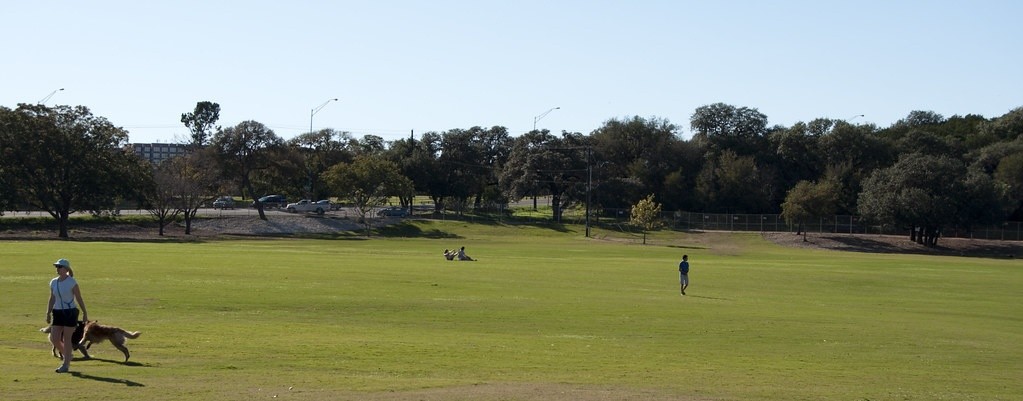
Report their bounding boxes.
[55,265,65,268]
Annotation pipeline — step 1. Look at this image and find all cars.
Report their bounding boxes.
[377,206,408,218]
[253,195,281,210]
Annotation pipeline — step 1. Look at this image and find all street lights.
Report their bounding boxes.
[534,106,560,132]
[310,99,339,147]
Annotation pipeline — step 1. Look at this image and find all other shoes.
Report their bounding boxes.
[681,293,685,295]
[62,351,72,361]
[55,364,68,373]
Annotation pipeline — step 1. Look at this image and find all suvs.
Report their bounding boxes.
[213,197,234,210]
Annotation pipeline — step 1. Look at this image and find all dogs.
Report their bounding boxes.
[39,320,142,363]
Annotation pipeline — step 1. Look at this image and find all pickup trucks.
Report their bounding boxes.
[287,200,327,214]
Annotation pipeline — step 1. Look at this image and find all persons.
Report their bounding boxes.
[46,259,87,372]
[458,246,477,261]
[679,255,689,295]
[444,248,458,260]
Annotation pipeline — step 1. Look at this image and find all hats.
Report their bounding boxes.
[53,259,69,270]
